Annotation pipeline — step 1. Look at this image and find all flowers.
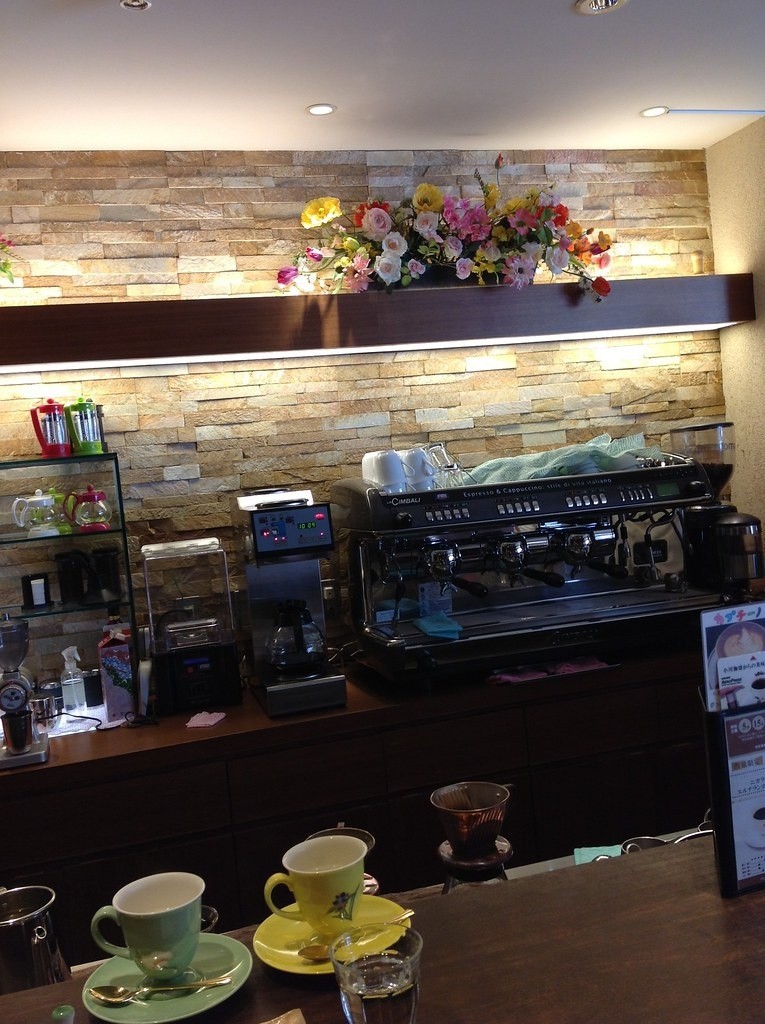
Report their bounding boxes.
[275,152,616,301]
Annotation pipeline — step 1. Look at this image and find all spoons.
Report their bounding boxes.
[297,908,415,961]
[88,976,232,1004]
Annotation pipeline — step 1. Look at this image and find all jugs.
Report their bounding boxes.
[30,397,72,458]
[64,397,104,455]
[12,483,114,538]
[0,885,71,997]
[264,598,327,678]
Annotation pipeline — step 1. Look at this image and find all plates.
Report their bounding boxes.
[80,932,253,1024]
[715,622,765,660]
[744,830,765,848]
[253,892,411,973]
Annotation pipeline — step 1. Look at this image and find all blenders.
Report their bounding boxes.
[0,613,51,770]
[137,539,252,722]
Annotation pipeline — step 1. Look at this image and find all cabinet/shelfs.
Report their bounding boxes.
[0,452,134,622]
[387,703,537,887]
[0,759,246,961]
[657,675,714,832]
[524,684,664,868]
[220,727,387,924]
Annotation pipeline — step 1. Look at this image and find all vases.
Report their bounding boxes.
[362,261,505,289]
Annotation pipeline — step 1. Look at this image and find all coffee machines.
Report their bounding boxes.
[669,420,765,608]
[228,487,349,720]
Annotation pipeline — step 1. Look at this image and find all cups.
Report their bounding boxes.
[263,835,368,948]
[753,807,765,836]
[0,710,33,755]
[431,780,510,856]
[750,677,765,698]
[328,922,423,1024]
[360,440,466,495]
[90,871,205,1000]
[28,696,55,730]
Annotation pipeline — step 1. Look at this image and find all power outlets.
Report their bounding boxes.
[175,596,201,622]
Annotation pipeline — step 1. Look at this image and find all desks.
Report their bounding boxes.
[0,665,390,788]
[0,825,765,1024]
